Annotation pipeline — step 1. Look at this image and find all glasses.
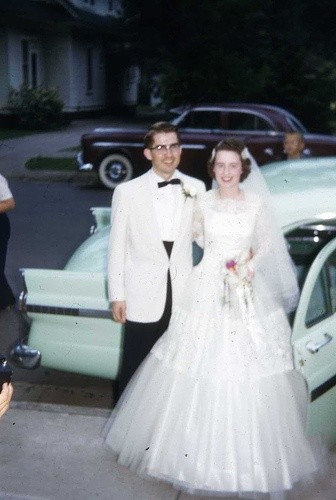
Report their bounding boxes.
[151,144,180,152]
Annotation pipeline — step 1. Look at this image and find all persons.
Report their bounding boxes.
[0,174,17,308]
[272,133,310,163]
[102,138,319,500]
[107,122,206,409]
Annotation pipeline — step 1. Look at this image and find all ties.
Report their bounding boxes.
[158,179,181,187]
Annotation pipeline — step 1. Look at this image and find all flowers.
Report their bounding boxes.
[224,258,238,271]
[182,183,197,204]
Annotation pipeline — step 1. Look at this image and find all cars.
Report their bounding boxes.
[10,155,336,395]
[75,102,336,192]
